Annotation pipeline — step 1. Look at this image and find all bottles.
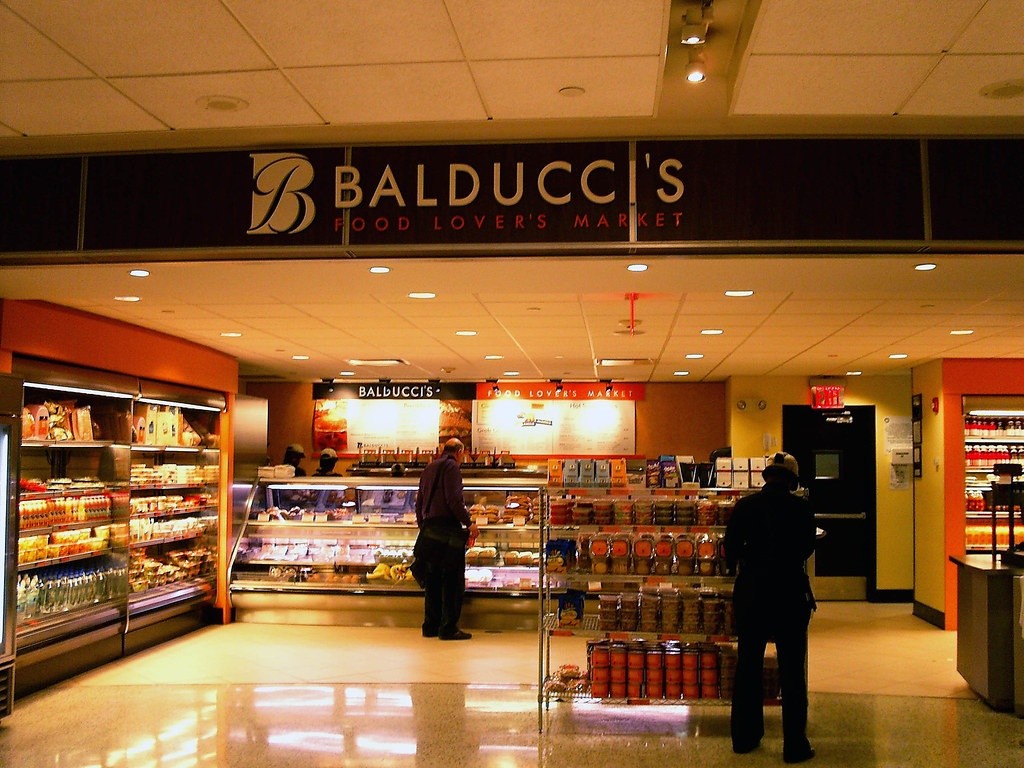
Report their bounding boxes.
[965,490,1021,512]
[966,415,1024,440]
[20,493,130,530]
[15,564,126,623]
[965,445,1024,467]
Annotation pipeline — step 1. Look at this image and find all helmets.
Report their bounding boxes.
[762,452,798,490]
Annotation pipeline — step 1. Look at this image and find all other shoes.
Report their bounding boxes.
[784,749,815,763]
[439,630,472,640]
[422,631,439,637]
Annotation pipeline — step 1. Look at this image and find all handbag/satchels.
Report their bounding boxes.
[413,518,470,571]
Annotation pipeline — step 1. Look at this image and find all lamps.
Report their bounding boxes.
[428,379,441,393]
[486,379,499,392]
[680,4,713,84]
[549,379,563,392]
[320,377,335,393]
[599,378,614,391]
[736,397,769,416]
[379,378,391,392]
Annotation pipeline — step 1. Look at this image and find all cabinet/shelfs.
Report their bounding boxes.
[537,487,808,734]
[128,442,220,600]
[16,439,130,636]
[230,476,546,593]
[965,437,1024,551]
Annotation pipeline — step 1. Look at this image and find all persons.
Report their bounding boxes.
[414,437,483,641]
[726,448,820,762]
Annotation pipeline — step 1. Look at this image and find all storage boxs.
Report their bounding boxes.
[717,458,767,488]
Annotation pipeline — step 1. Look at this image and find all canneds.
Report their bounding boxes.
[591,648,720,701]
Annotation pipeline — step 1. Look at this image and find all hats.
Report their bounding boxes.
[320,448,338,459]
[287,444,306,458]
